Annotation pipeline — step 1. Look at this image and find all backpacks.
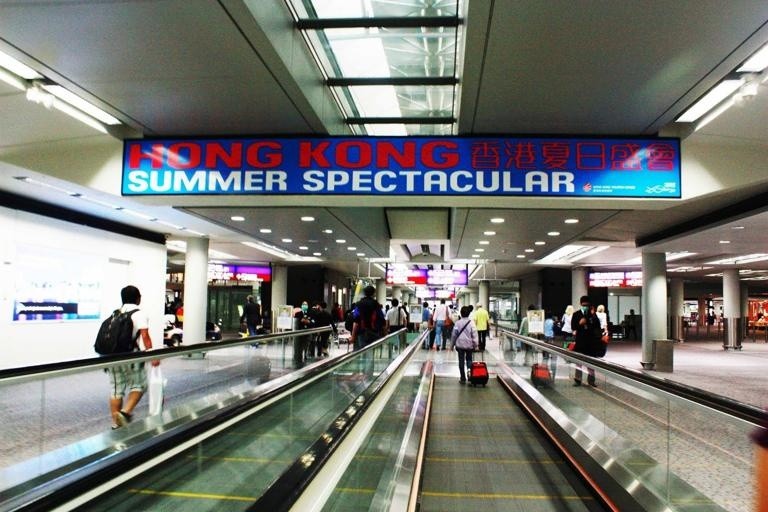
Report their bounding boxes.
[94,309,140,355]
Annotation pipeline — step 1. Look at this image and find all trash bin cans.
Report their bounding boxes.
[651,339,673,374]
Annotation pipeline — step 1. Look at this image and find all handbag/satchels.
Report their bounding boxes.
[445,319,452,326]
[601,331,609,343]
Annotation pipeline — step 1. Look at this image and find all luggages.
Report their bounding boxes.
[471,349,488,387]
[531,350,551,389]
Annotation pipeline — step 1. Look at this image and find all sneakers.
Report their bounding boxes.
[112,410,132,430]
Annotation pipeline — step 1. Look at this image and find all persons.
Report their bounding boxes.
[99,285,161,431]
[450,306,480,384]
[379,298,414,351]
[624,310,638,341]
[238,294,261,347]
[294,301,355,367]
[561,295,608,388]
[518,304,562,364]
[421,298,489,352]
[348,286,386,378]
